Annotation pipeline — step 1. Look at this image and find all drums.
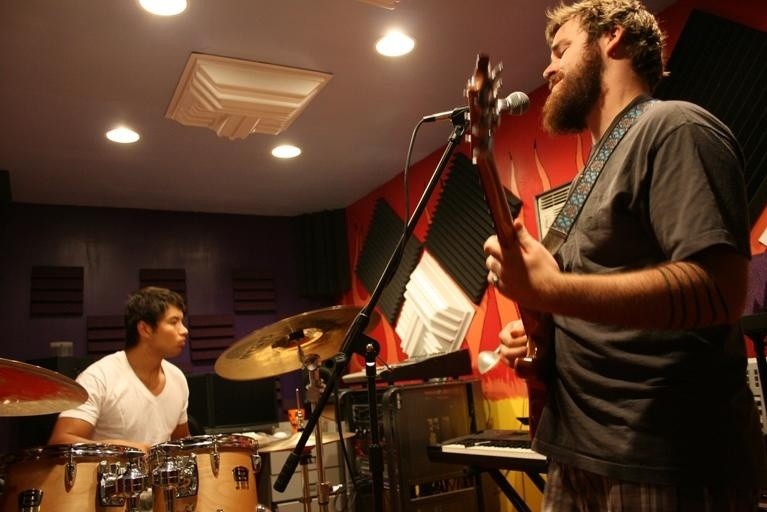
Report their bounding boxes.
[147,435,261,512]
[3,443,143,512]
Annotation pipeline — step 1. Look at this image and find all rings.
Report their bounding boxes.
[492,274,498,285]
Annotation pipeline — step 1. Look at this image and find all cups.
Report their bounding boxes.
[289,408,304,432]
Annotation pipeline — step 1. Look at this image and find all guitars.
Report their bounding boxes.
[466,51,544,440]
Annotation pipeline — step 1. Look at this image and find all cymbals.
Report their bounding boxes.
[1,358,88,418]
[257,430,356,452]
[215,305,381,381]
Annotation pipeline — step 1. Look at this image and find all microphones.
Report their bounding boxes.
[422,91,531,123]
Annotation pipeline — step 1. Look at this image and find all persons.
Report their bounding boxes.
[478,1,766,512]
[48,284,193,456]
